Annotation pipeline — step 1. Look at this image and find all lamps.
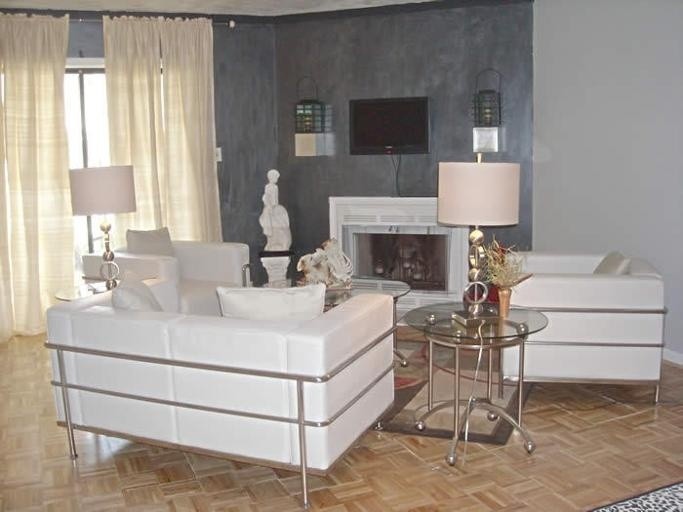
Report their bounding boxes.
[437,162,521,327]
[68,165,136,290]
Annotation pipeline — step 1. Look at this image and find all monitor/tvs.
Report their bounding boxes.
[349,96,431,155]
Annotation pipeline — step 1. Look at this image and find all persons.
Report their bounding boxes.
[255,168,292,252]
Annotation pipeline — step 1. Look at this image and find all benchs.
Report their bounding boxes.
[47,273,396,507]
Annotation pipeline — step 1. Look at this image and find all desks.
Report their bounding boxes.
[323,279,411,368]
[403,302,549,466]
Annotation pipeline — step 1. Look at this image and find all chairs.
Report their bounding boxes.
[500,244,667,429]
[82,238,256,316]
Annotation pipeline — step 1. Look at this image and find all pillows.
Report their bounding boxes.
[125,227,181,276]
[111,270,165,313]
[215,282,326,322]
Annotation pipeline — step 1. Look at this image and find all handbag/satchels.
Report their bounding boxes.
[296,238,354,290]
